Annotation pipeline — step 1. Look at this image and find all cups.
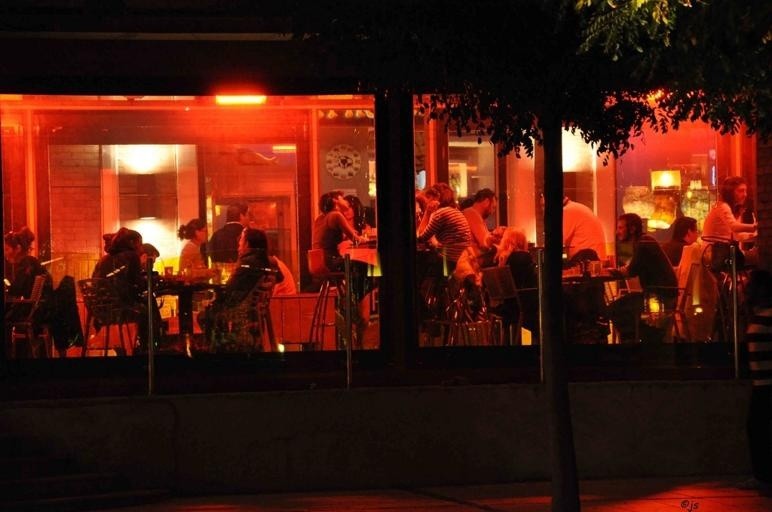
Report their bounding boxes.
[165,266,173,277]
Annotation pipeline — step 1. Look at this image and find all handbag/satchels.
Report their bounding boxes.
[700,237,746,273]
[54,323,83,352]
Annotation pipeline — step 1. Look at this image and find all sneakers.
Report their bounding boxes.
[341,306,363,326]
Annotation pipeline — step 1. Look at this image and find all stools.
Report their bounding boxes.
[1,242,282,348]
[337,243,771,351]
[307,247,350,352]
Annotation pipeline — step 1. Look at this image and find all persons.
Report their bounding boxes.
[87,201,281,354]
[3,227,55,334]
[415,183,543,339]
[312,191,374,334]
[563,174,758,344]
